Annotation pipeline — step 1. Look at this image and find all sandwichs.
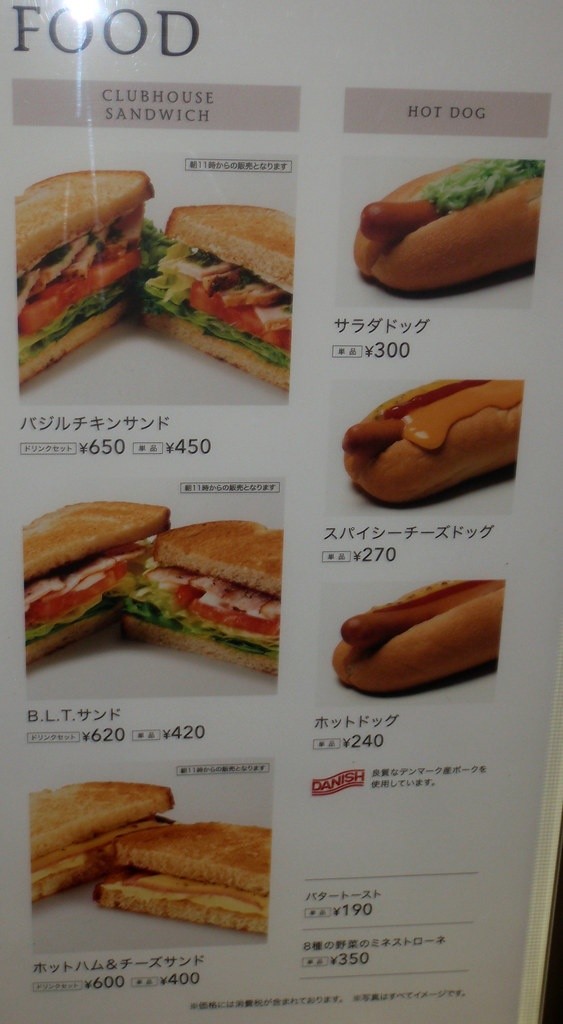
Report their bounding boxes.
[11,167,301,934]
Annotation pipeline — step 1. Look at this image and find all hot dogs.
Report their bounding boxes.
[330,157,539,699]
